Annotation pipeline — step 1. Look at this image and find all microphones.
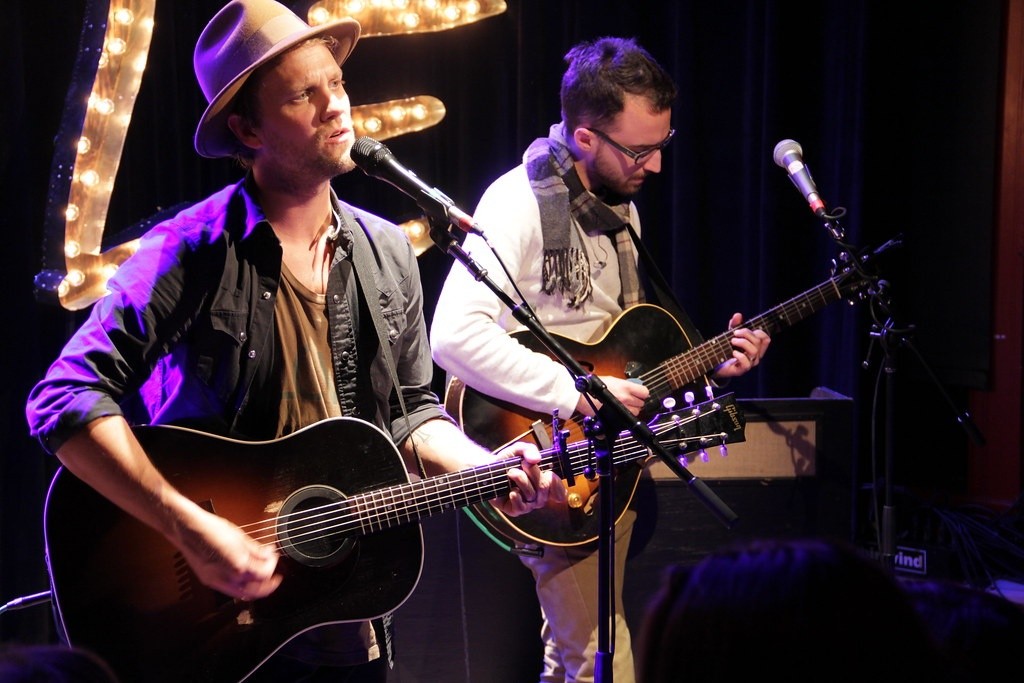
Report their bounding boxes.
[773,138,826,218]
[350,136,484,237]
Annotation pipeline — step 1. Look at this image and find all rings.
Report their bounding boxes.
[239,585,246,601]
[526,498,537,502]
[750,356,757,361]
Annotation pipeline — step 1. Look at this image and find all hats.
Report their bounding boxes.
[193,0,361,160]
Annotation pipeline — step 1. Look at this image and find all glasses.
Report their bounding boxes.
[586,125,675,164]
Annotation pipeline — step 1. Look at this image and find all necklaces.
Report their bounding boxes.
[588,234,608,268]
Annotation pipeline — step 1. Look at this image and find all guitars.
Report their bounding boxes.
[440,233,914,550]
[41,383,749,682]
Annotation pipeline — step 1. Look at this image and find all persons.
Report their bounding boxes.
[428,38,771,683]
[24,0,568,683]
